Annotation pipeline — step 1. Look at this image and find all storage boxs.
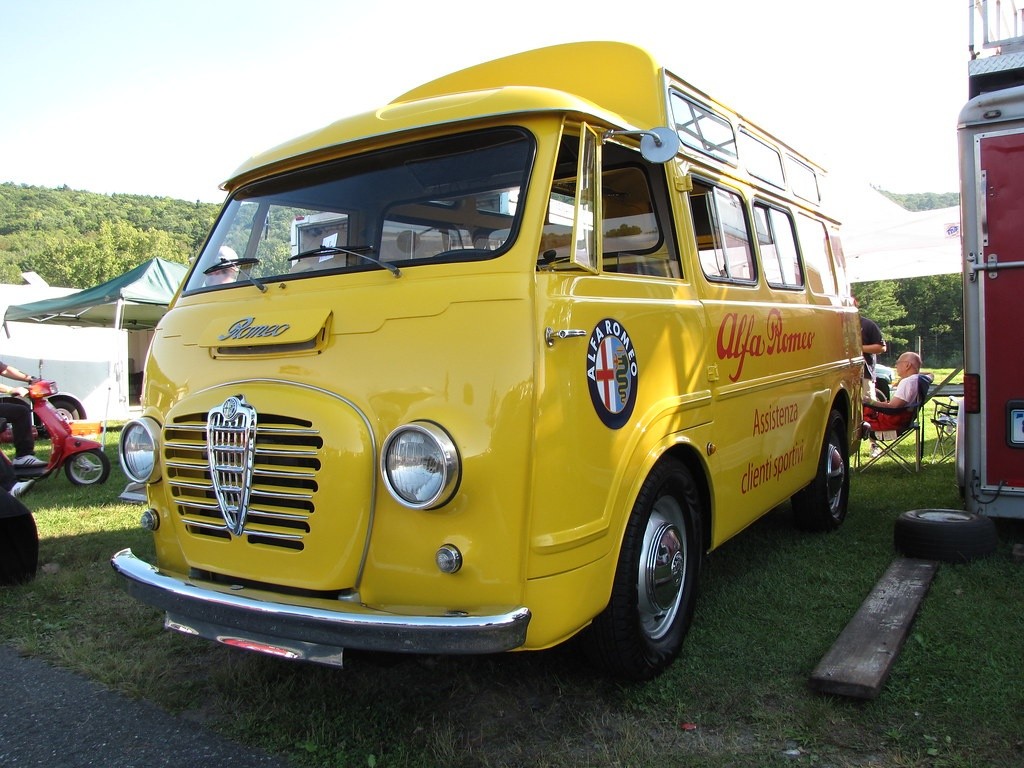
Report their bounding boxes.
[68,420,102,436]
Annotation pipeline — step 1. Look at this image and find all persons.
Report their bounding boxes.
[862,352,921,430]
[852,298,889,457]
[0,361,48,500]
[189,246,238,286]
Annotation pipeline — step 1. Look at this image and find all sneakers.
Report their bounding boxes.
[869,447,879,458]
[12,454,49,465]
[8,479,35,498]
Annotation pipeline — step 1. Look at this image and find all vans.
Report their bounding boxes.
[107,37,867,691]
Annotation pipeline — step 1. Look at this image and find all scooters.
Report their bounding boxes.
[1,360,111,487]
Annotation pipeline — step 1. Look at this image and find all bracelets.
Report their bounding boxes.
[24,374,28,381]
[865,399,875,405]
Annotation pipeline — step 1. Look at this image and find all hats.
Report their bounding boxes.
[189,246,240,271]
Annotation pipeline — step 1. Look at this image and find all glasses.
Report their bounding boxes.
[896,359,914,367]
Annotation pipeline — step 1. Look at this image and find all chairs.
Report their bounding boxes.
[854,372,959,474]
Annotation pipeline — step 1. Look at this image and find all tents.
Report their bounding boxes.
[3,257,189,451]
